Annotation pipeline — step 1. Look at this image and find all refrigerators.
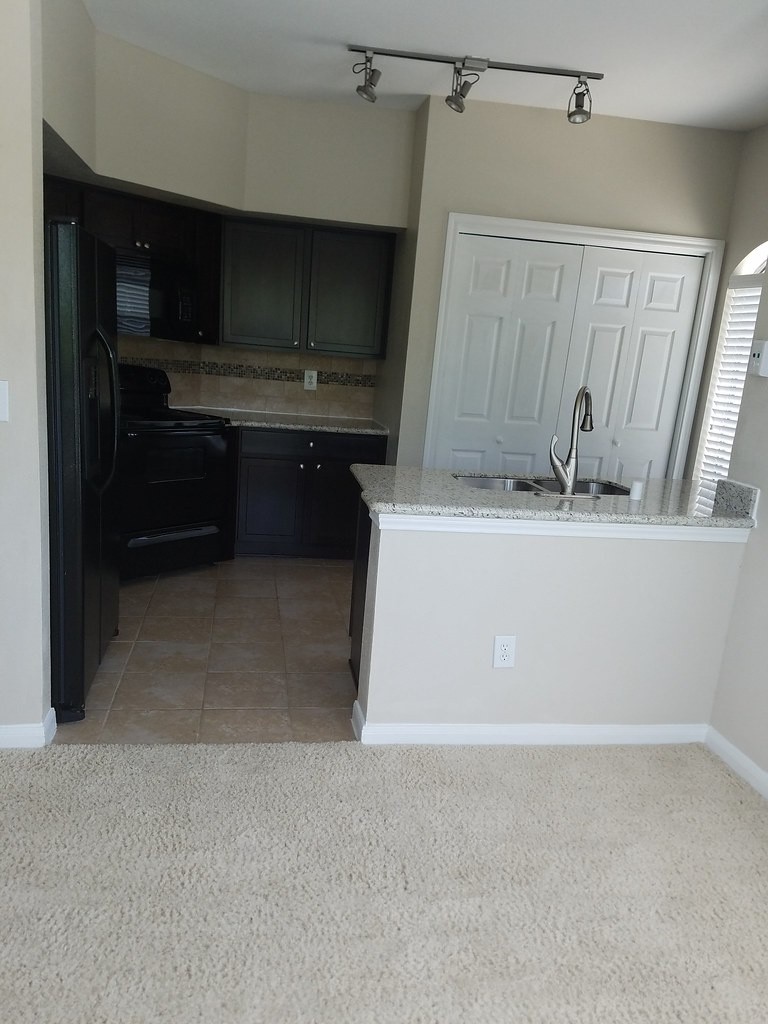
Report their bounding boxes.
[44,213,121,725]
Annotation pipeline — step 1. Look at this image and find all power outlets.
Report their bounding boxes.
[493,636,517,668]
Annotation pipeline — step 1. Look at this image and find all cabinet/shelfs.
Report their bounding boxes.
[234,417,393,559]
[220,216,398,358]
[83,183,222,348]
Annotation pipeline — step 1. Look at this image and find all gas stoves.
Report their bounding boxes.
[115,362,231,433]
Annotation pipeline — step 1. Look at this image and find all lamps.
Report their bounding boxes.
[347,43,604,125]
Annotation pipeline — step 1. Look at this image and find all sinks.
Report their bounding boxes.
[533,478,631,496]
[451,473,545,492]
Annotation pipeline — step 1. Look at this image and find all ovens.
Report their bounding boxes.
[118,428,234,580]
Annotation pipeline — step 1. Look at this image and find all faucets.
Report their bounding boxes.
[550,386,593,495]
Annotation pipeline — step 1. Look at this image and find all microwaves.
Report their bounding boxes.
[117,245,220,349]
